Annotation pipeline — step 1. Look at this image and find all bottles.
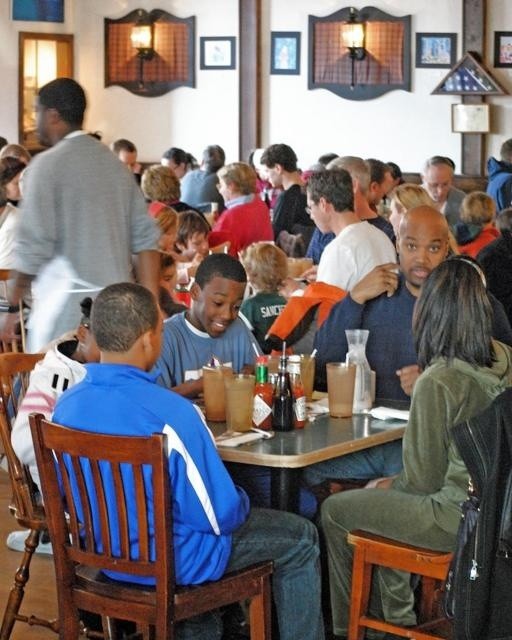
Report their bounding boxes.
[253,355,304,430]
[346,329,372,415]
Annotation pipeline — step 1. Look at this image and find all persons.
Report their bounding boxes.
[280,206,509,516]
[304,156,395,265]
[366,139,511,318]
[49,282,325,635]
[110,140,341,319]
[10,298,101,513]
[149,253,316,524]
[240,242,301,350]
[0,80,160,554]
[1,138,30,315]
[275,167,399,302]
[321,257,510,637]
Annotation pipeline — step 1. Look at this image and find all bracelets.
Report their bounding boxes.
[2,305,19,313]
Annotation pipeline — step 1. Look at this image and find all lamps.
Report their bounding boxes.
[130,7,155,94]
[340,6,367,95]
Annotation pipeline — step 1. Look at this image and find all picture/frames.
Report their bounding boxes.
[269,30,301,76]
[199,35,237,70]
[415,32,457,68]
[493,30,512,68]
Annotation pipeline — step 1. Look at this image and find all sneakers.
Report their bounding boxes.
[6,529,53,555]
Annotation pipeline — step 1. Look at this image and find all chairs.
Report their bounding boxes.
[275,230,297,257]
[346,387,511,639]
[1,269,30,353]
[1,352,275,640]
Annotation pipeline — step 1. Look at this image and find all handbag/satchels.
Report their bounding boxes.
[443,480,481,627]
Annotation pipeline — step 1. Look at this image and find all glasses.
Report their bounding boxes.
[305,206,311,215]
[216,183,223,190]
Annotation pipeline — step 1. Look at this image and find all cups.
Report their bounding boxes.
[302,356,315,403]
[203,366,254,432]
[326,362,356,418]
